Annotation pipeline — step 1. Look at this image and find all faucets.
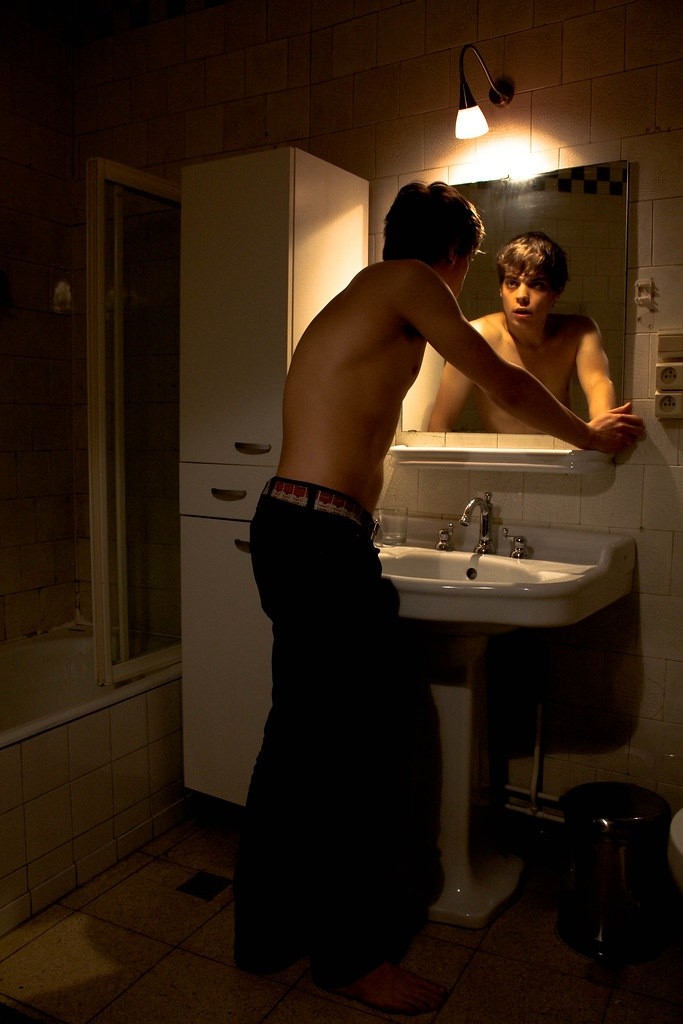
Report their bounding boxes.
[460,491,493,554]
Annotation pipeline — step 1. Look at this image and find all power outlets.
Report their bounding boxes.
[653,392,683,420]
[653,362,683,391]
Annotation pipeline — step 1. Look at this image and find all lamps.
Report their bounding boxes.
[454,43,512,142]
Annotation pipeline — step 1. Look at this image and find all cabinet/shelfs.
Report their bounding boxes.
[172,149,369,814]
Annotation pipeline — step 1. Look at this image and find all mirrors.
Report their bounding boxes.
[414,157,630,430]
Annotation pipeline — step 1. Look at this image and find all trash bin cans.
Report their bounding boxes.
[556,782,671,959]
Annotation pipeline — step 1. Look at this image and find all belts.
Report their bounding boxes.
[259,477,383,543]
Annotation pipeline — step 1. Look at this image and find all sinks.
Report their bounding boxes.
[366,546,599,627]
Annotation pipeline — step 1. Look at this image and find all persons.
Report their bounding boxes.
[230,181,647,1014]
[427,230,615,435]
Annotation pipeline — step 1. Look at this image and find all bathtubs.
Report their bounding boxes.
[1,625,200,939]
